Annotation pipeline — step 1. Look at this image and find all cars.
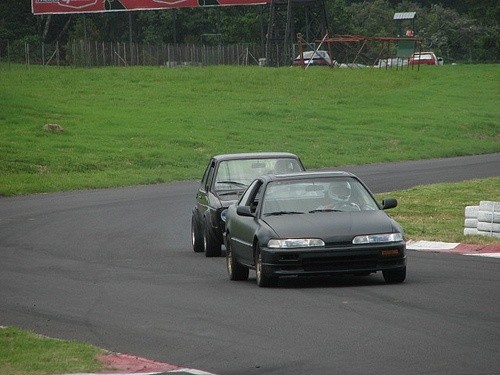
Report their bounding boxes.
[225,171,407,287]
[190,152,307,258]
[408,51,442,65]
[294,50,333,65]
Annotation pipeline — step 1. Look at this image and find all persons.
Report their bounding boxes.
[317,182,361,211]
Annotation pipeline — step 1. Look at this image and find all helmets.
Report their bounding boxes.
[327,180,351,203]
